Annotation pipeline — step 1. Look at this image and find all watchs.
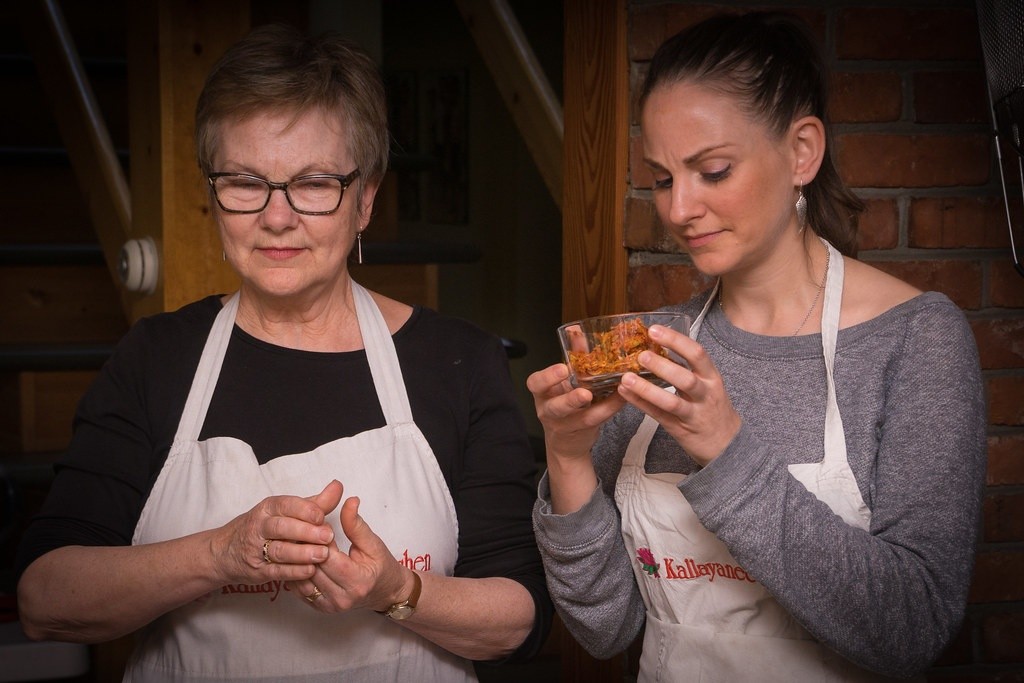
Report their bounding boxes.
[375,571,423,621]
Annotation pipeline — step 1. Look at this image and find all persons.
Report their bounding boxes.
[526,7,986,683]
[9,21,554,683]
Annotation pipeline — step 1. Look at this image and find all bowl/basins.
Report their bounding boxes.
[557,311,691,403]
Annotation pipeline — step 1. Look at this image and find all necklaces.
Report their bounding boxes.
[718,241,829,335]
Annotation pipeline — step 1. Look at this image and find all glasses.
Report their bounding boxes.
[208,168,361,216]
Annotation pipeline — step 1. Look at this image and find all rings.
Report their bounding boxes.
[305,586,321,603]
[263,540,272,563]
